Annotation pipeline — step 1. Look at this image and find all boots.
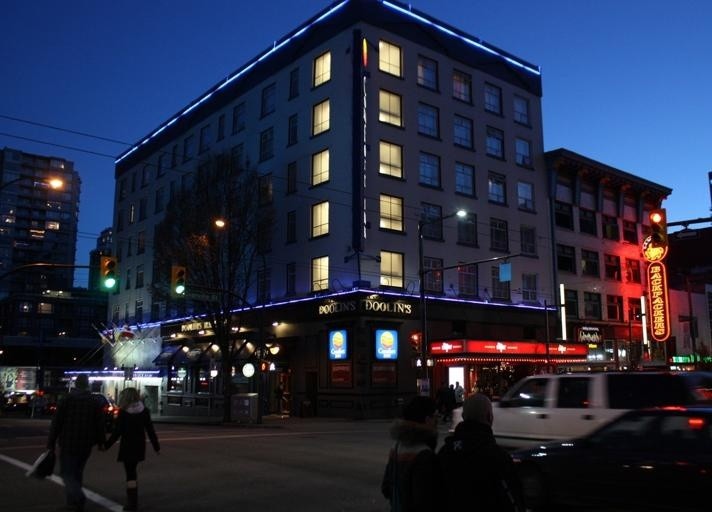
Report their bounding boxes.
[123,488,138,512]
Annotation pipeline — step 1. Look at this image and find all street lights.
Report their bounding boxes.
[1,176,64,190]
[415,208,467,395]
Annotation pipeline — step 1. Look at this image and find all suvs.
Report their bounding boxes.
[449,368,711,452]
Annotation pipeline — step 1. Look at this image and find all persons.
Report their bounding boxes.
[429,391,528,512]
[99,387,163,512]
[43,372,108,511]
[435,375,513,424]
[274,382,286,411]
[381,396,439,512]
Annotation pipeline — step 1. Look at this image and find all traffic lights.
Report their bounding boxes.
[648,209,669,248]
[101,256,119,294]
[260,361,270,373]
[425,356,434,368]
[415,357,424,368]
[169,266,186,298]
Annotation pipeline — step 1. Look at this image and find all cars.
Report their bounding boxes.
[1,389,120,435]
[499,405,711,511]
